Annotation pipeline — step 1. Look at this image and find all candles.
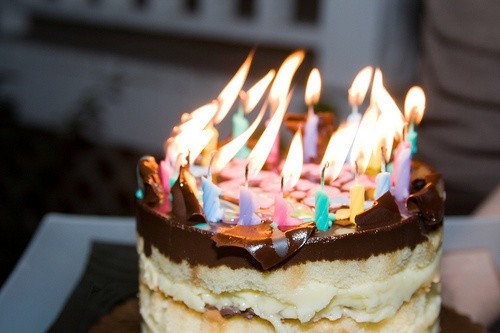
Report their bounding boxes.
[304,68,322,162]
[239,99,284,222]
[203,105,265,223]
[273,128,302,228]
[346,65,427,216]
[162,48,305,203]
[348,105,378,226]
[313,124,356,231]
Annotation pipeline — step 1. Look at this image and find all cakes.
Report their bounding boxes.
[131,155,445,333]
